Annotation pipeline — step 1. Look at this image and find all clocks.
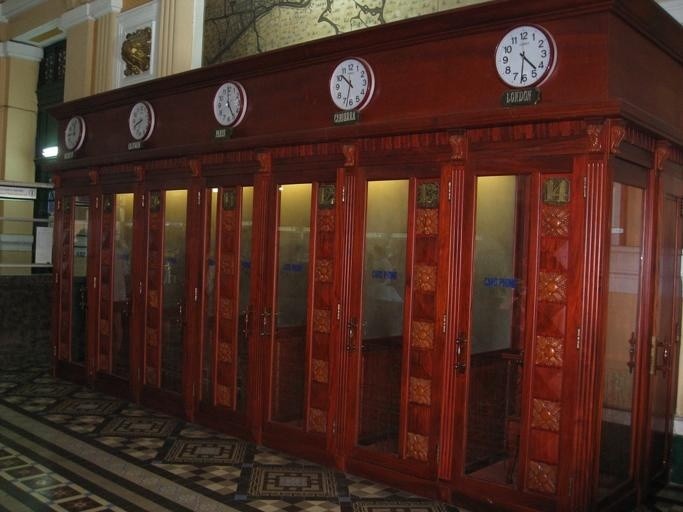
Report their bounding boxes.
[494,25,559,90]
[128,100,155,142]
[64,115,86,152]
[328,56,376,112]
[212,80,248,130]
